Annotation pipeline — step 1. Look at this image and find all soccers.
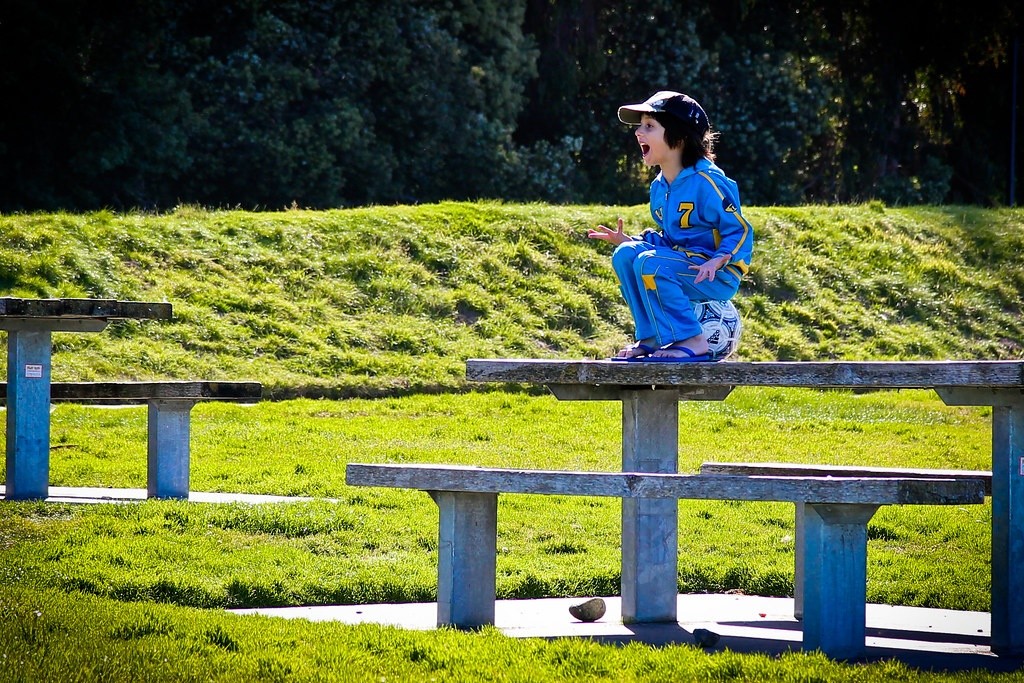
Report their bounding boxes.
[681,300,741,363]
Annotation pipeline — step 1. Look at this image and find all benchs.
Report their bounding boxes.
[697,462,994,622]
[346,461,986,663]
[0,380,261,502]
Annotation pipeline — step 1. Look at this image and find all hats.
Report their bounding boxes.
[618,91,709,126]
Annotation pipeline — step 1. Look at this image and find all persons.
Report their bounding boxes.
[588,89,753,362]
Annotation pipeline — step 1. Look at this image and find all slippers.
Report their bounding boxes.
[643,346,709,362]
[611,344,664,362]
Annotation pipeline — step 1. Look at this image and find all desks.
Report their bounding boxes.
[0,298,172,500]
[466,358,1024,658]
